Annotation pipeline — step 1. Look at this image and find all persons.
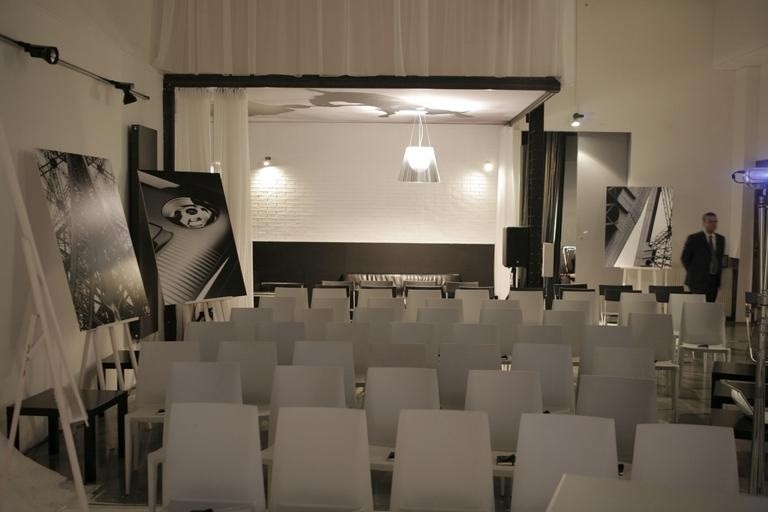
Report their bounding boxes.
[680,212,725,302]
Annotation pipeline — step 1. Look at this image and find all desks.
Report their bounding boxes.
[98,350,140,390]
[710,408,768,441]
[8,385,128,484]
[711,379,768,409]
[711,361,768,396]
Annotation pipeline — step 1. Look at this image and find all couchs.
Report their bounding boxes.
[341,273,463,287]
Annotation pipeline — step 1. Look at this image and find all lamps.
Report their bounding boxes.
[26,45,60,64]
[398,108,440,184]
[114,81,138,106]
[571,112,585,127]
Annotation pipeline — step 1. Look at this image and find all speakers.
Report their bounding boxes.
[502,226,530,268]
[542,242,555,277]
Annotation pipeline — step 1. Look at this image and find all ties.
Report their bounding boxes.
[708,235,719,276]
